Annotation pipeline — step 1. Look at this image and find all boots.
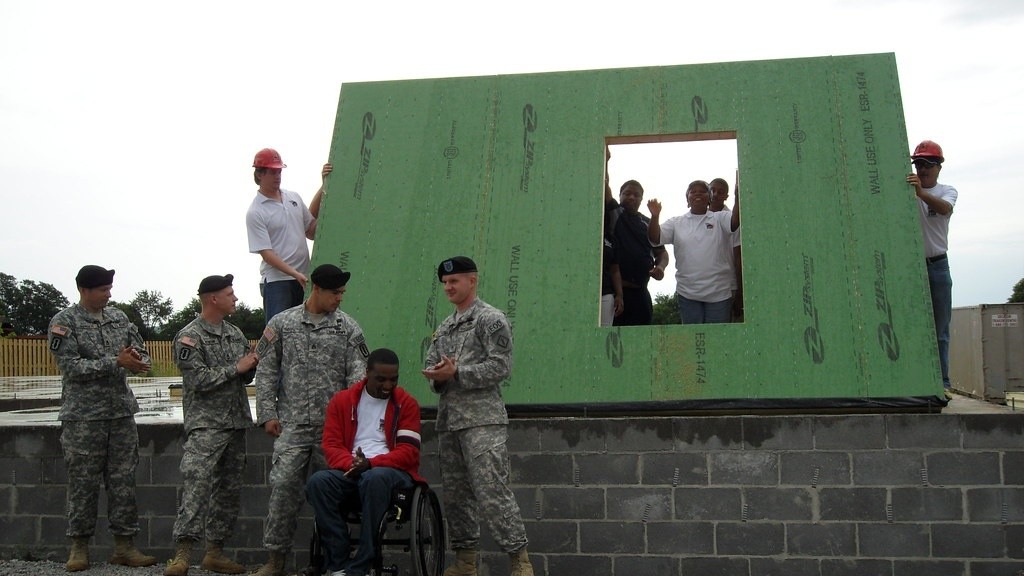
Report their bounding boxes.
[112,535,156,567]
[249,551,286,576]
[510,547,533,576]
[164,541,192,576]
[67,536,89,571]
[443,550,477,576]
[202,540,245,573]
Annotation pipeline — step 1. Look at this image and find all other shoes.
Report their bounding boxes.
[945,387,952,398]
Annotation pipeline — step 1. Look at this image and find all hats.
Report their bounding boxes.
[197,274,234,295]
[438,256,478,282]
[76,265,115,288]
[912,157,941,165]
[311,264,351,289]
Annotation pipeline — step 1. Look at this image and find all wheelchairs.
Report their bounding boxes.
[309,482,446,576]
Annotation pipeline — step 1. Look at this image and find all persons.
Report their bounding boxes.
[256,264,371,576]
[247,148,317,325]
[906,140,958,400]
[305,348,421,576]
[163,274,258,576]
[309,163,332,220]
[601,148,744,325]
[47,265,157,571]
[422,256,533,576]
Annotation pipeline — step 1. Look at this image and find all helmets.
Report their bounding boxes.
[911,140,944,162]
[252,148,284,168]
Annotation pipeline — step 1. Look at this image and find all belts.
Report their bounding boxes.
[927,253,947,263]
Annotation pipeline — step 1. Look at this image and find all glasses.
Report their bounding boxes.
[915,160,938,169]
